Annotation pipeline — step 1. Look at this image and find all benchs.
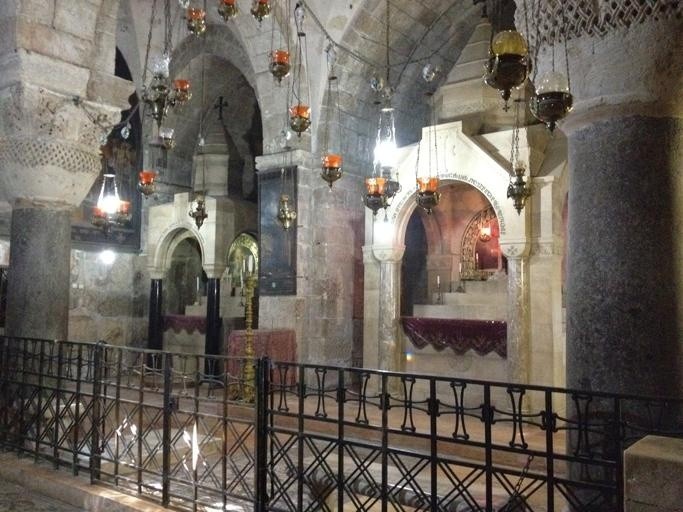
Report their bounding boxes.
[287,452,526,512]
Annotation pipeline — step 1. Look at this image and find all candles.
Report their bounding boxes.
[437,275,440,285]
[459,263,462,272]
[476,252,479,262]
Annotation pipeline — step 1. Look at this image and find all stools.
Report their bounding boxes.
[12,398,85,450]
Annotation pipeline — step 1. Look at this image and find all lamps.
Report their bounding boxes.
[312,77,352,191]
[218,0,314,238]
[480,1,574,220]
[359,111,408,217]
[410,88,452,218]
[92,0,217,241]
